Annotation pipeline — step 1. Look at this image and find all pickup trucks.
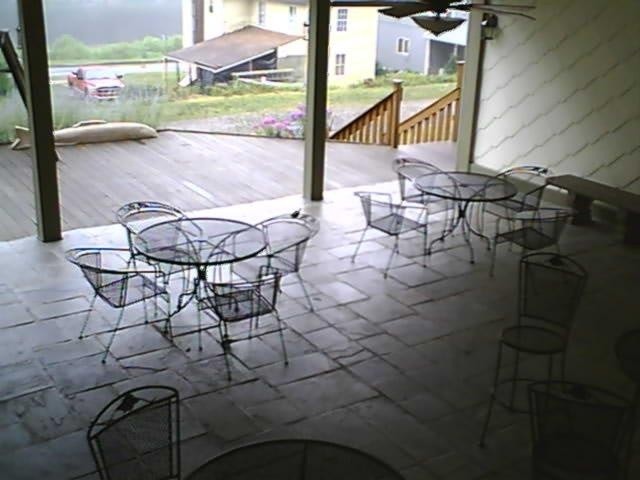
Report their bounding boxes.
[66,65,125,102]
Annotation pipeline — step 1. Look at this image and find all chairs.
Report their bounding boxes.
[477,252,587,448]
[86,385,180,480]
[480,166,554,251]
[115,200,203,324]
[194,265,289,381]
[352,191,429,280]
[490,209,580,278]
[527,380,632,480]
[66,248,173,364]
[392,156,459,242]
[231,212,321,326]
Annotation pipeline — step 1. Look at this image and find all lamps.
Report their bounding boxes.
[480,15,499,40]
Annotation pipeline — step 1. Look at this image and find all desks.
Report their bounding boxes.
[414,172,518,263]
[134,218,269,353]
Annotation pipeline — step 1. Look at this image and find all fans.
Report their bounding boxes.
[330,0,536,36]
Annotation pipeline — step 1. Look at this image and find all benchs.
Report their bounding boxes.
[546,174,640,245]
